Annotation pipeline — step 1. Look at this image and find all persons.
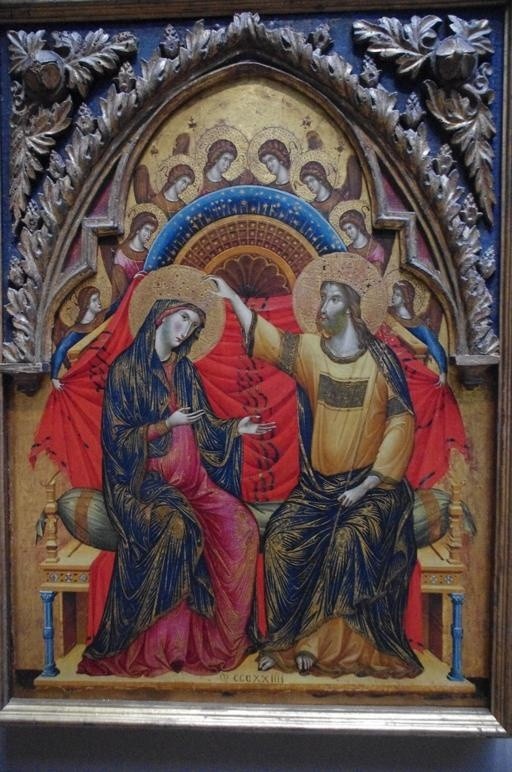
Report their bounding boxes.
[339,209,383,273]
[201,273,414,671]
[110,211,160,308]
[151,165,195,214]
[391,280,448,389]
[202,140,236,195]
[258,139,290,192]
[299,161,343,213]
[49,284,103,392]
[102,298,278,675]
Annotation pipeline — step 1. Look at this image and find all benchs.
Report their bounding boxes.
[35,474,467,681]
